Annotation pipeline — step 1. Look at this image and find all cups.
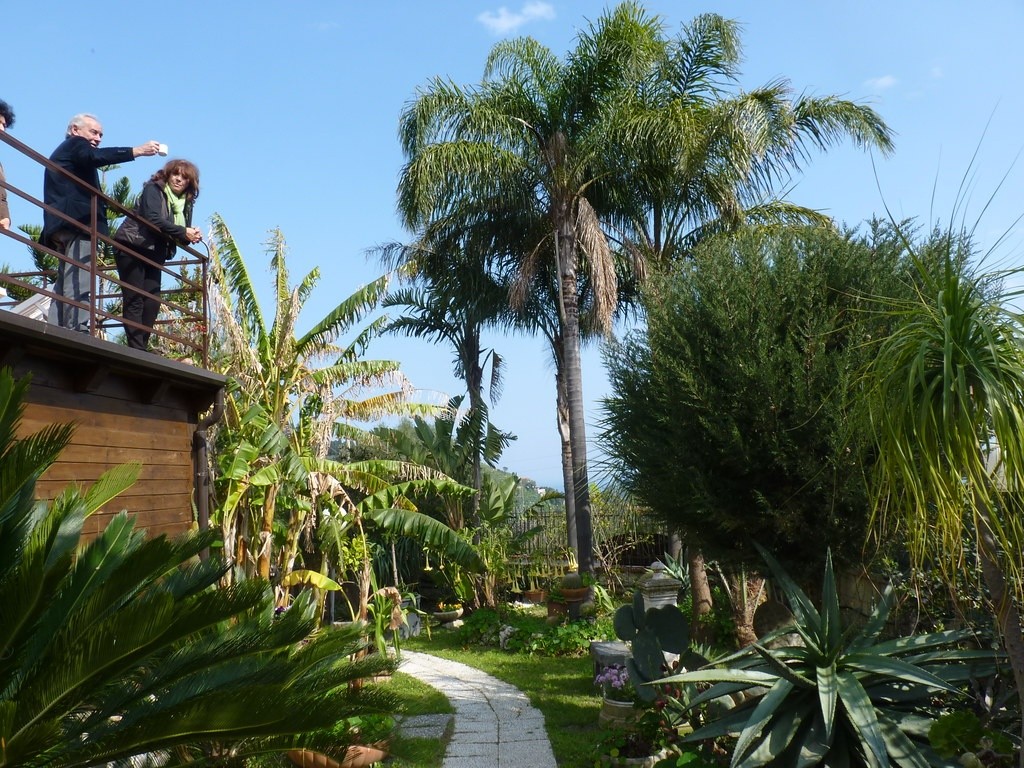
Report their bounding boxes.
[158,144,168,156]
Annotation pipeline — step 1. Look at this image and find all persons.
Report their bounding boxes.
[0,99,16,231]
[37,113,160,333]
[112,159,202,353]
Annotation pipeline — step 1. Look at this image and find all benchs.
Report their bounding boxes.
[590,641,633,682]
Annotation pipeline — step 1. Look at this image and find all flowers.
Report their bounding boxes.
[595,663,635,702]
[437,602,461,612]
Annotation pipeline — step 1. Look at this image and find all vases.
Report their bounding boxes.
[433,609,464,628]
[599,699,634,732]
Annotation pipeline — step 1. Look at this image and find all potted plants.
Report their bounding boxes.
[372,576,420,642]
[545,573,595,618]
